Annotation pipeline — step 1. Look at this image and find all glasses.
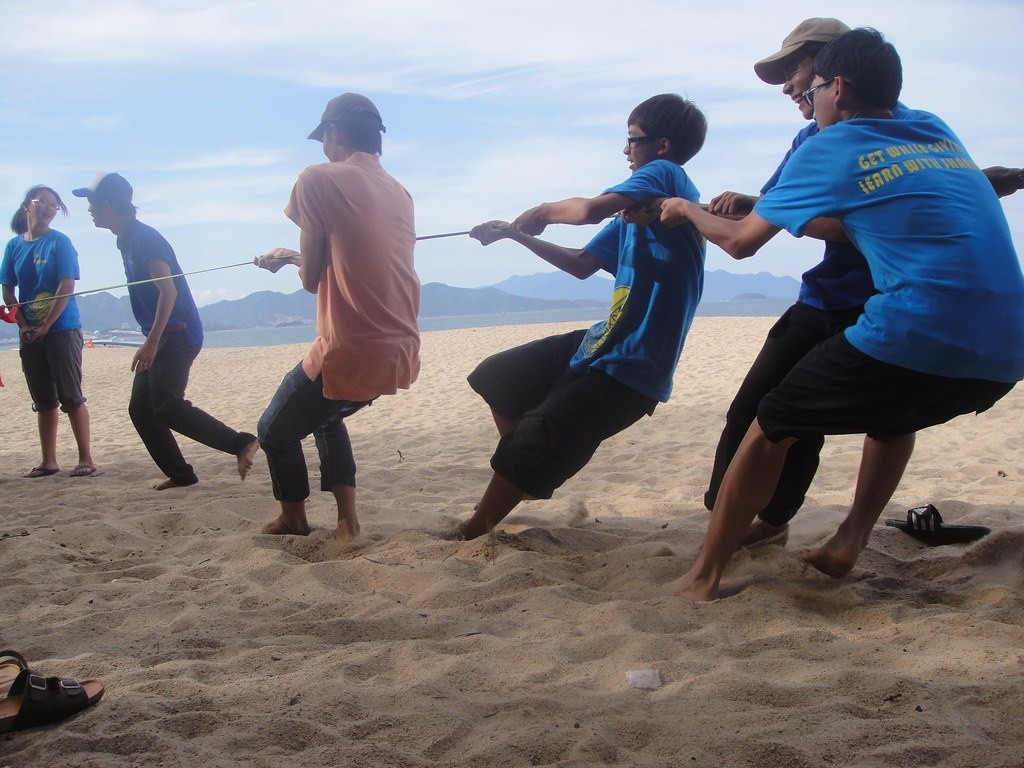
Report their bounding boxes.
[802,77,835,107]
[625,134,651,148]
[32,198,60,210]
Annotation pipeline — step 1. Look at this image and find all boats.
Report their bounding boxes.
[83,339,145,347]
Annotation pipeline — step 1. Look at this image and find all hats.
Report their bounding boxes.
[72,173,133,202]
[308,93,386,143]
[754,17,851,85]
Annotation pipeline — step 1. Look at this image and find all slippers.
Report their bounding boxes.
[884,503,993,546]
[24,467,59,477]
[71,465,98,477]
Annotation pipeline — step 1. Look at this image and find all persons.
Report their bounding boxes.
[444,93,708,542]
[622,17,1024,605]
[71,170,259,491]
[254,93,421,543]
[0,185,98,478]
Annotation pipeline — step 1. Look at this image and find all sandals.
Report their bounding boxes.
[0,649,107,742]
